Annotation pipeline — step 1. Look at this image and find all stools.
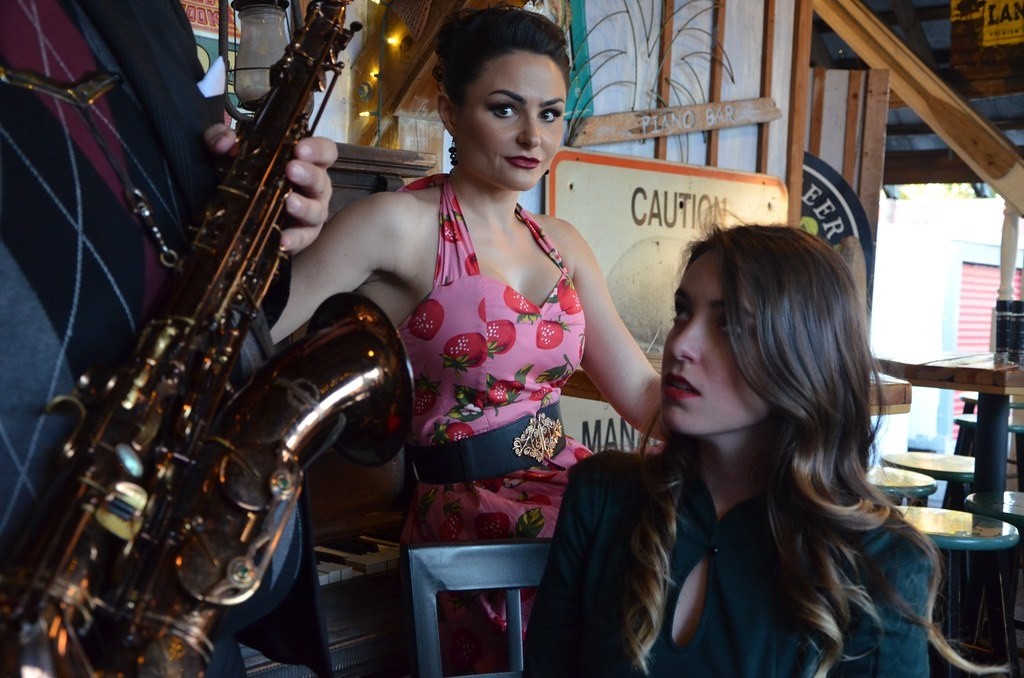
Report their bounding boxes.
[867,467,938,507]
[893,491,1024,678]
[879,452,975,511]
[942,413,1024,511]
[960,391,1024,414]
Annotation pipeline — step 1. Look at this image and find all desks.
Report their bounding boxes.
[873,351,1024,491]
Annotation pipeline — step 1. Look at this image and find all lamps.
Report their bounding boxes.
[218,0,314,136]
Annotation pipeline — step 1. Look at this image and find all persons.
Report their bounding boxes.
[516,222,1009,678]
[268,6,672,678]
[0,0,342,678]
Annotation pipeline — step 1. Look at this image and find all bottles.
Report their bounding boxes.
[1007,301,1024,364]
[996,300,1011,353]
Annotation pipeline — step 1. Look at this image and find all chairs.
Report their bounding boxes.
[406,538,550,678]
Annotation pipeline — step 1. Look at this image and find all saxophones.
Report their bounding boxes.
[0,2,417,678]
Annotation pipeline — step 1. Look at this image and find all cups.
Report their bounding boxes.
[994,353,1009,367]
